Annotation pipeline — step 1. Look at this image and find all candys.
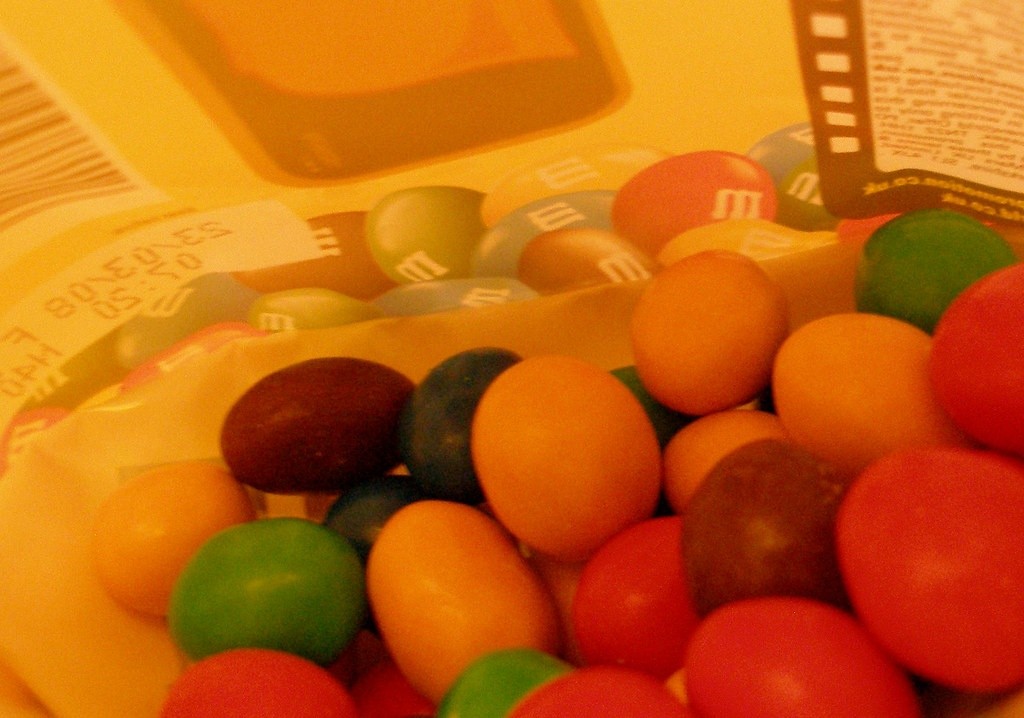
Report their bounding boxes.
[79,210,1024,718]
[0,121,853,473]
